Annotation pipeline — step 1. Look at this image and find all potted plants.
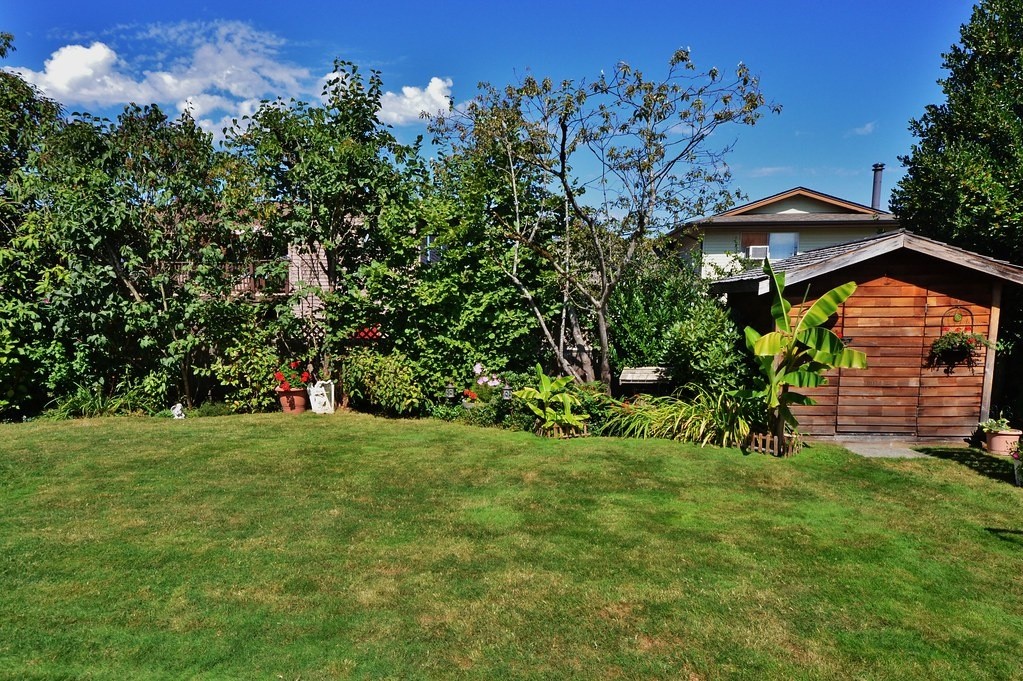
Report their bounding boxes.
[978,411,1023,456]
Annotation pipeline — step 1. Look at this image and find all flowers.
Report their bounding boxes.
[273,359,312,391]
[1005,440,1023,460]
[462,362,503,403]
[930,326,1015,363]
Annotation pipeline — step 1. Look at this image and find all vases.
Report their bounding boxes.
[1014,460,1023,486]
[463,400,487,410]
[275,386,306,414]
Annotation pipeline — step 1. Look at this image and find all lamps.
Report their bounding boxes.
[445,383,458,399]
[501,379,514,401]
